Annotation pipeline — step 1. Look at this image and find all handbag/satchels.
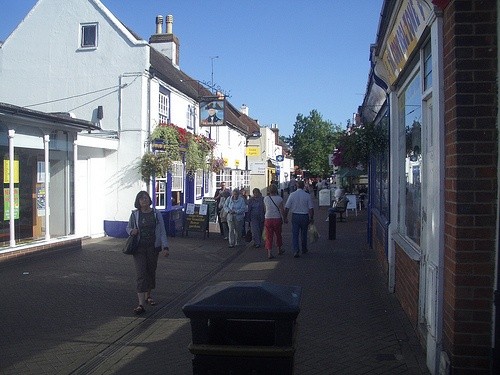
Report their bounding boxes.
[281,215,283,225]
[246,226,252,243]
[122,213,140,255]
[309,223,321,244]
[221,195,231,223]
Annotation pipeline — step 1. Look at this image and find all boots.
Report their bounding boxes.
[267,250,273,259]
[277,247,286,255]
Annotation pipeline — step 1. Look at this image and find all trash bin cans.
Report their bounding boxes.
[180,278,304,375]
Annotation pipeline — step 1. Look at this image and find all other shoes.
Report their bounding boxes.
[223,237,228,240]
[235,244,240,247]
[254,242,261,248]
[301,249,309,254]
[293,252,300,258]
[228,244,234,248]
[261,241,265,248]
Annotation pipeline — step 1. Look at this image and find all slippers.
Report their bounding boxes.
[135,304,144,314]
[145,296,157,305]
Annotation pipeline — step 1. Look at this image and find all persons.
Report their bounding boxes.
[213,176,367,259]
[126,191,170,315]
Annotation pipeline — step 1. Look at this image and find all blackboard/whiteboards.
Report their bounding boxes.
[202,196,218,223]
[184,203,209,232]
